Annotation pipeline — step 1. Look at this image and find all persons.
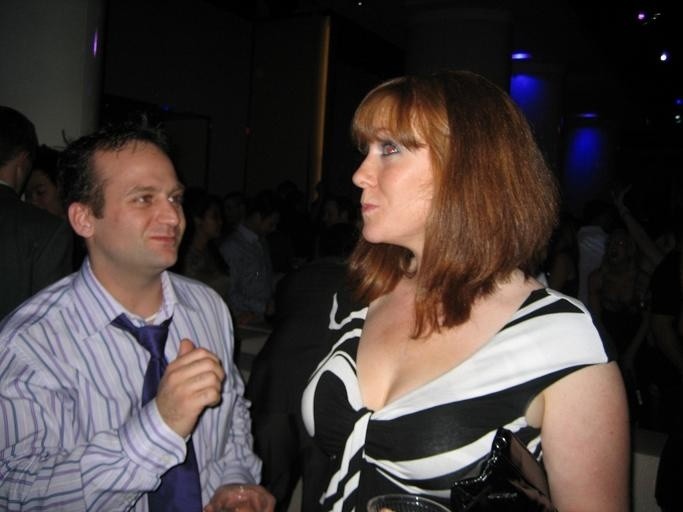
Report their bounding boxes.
[187,179,675,443]
[0,105,73,325]
[23,142,66,217]
[281,70,632,512]
[0,126,280,511]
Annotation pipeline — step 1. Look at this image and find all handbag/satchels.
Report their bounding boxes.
[447,424,558,512]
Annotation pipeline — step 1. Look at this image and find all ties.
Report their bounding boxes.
[108,311,202,512]
